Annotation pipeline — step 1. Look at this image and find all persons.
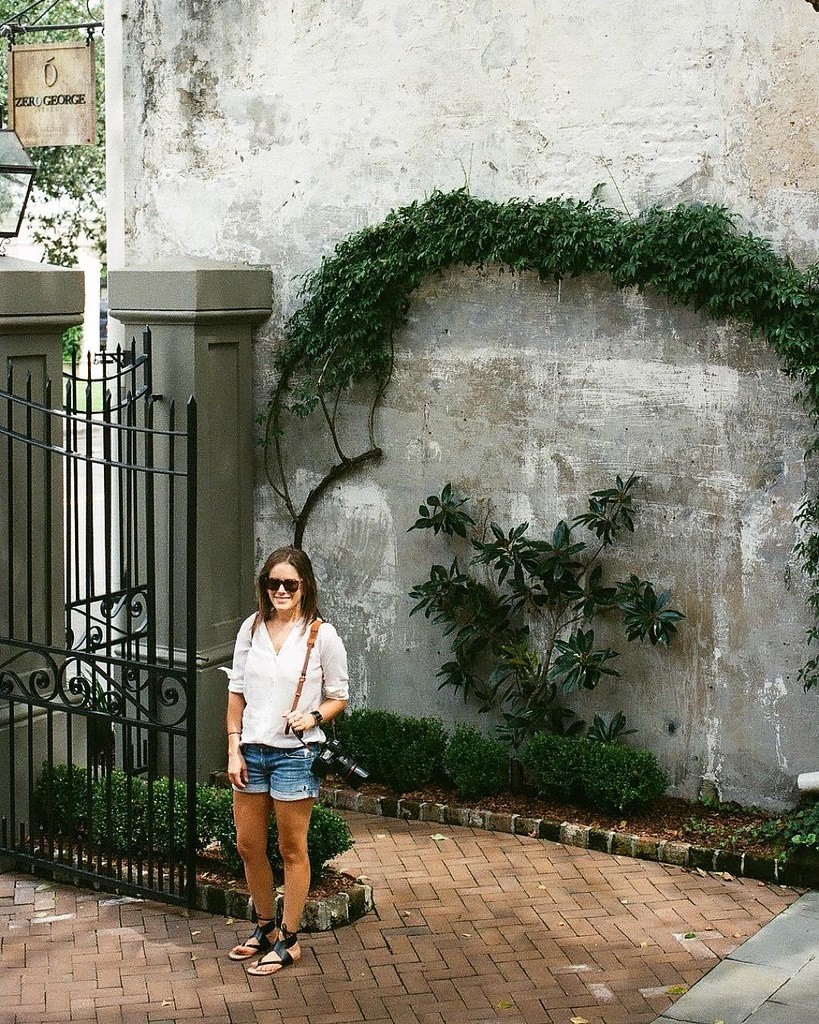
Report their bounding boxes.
[218,547,349,977]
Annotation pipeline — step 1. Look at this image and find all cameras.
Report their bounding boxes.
[311,738,370,790]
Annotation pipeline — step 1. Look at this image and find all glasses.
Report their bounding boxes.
[265,577,304,593]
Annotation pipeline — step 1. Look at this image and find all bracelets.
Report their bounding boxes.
[310,711,323,728]
[227,732,241,735]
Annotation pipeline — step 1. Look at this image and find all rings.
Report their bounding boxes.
[293,716,296,722]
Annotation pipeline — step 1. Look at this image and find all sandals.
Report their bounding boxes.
[229,913,279,961]
[247,923,301,976]
